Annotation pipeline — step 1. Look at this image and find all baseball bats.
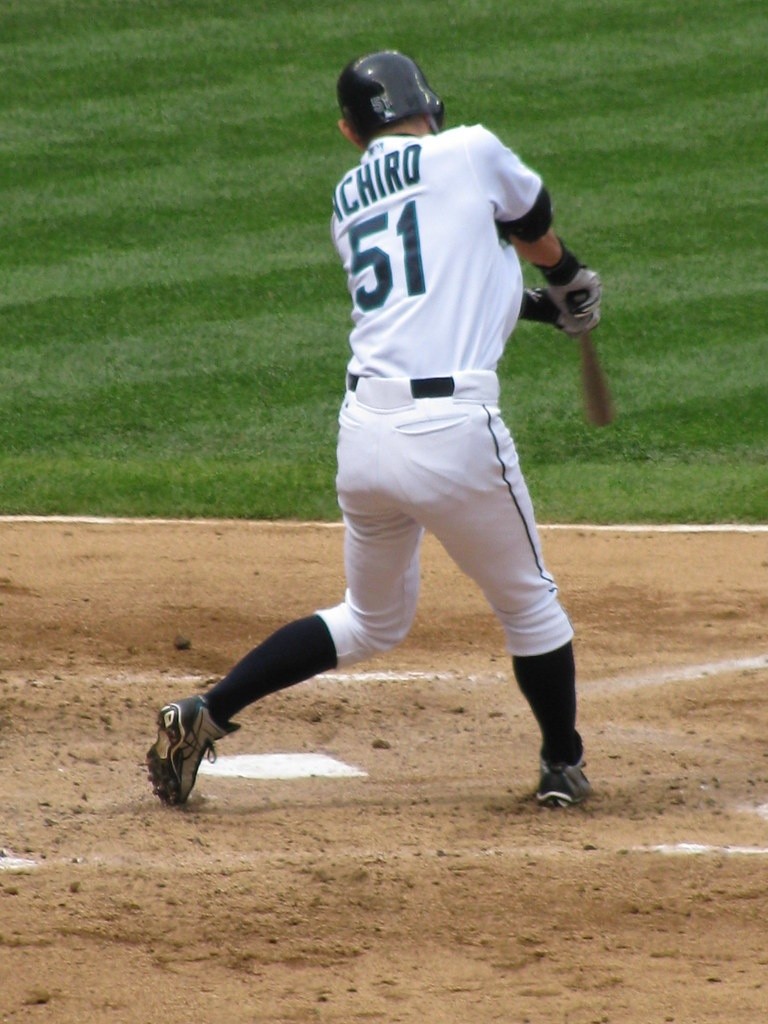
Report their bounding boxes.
[564,289,616,428]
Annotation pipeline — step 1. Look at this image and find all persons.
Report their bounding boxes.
[150,50,603,808]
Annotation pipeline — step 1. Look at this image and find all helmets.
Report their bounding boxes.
[336,50,444,143]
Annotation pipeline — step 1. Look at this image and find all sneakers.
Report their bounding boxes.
[145,693,243,805]
[536,747,592,805]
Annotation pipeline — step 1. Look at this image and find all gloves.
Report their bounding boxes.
[532,238,602,317]
[518,287,601,333]
